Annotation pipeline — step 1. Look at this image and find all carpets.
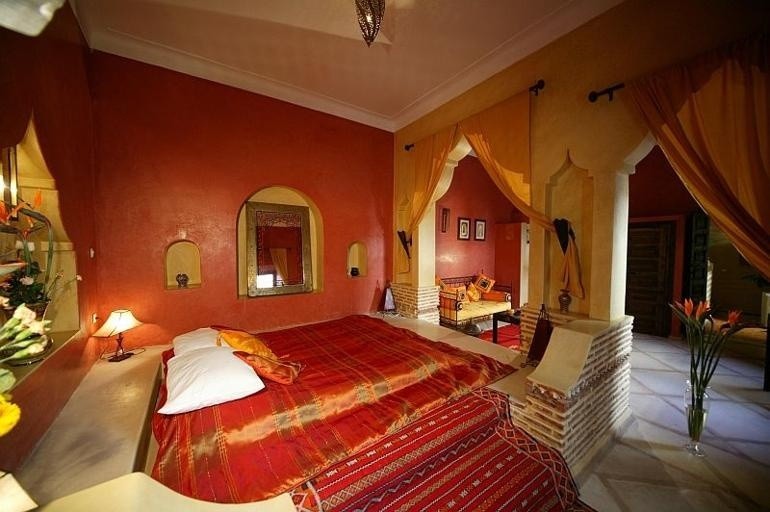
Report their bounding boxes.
[285,385,597,512]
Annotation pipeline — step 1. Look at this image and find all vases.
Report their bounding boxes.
[0,299,51,366]
[684,381,712,457]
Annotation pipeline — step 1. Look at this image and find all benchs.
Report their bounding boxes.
[439,275,511,330]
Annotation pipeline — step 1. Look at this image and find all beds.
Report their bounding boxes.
[146,312,518,501]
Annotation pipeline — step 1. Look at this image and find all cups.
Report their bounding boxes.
[507,309,514,316]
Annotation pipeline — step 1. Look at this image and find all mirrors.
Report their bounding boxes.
[245,201,314,298]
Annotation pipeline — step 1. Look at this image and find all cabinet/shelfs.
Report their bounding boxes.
[496,222,529,308]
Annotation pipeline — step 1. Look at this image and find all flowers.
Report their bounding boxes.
[0,302,51,438]
[0,188,83,305]
[668,298,743,440]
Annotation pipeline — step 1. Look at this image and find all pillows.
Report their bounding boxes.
[233,351,307,385]
[173,327,217,356]
[217,330,280,360]
[435,275,511,302]
[157,346,266,415]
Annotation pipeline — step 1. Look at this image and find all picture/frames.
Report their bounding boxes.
[474,219,486,241]
[457,217,470,240]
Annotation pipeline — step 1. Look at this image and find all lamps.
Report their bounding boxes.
[90,309,145,362]
